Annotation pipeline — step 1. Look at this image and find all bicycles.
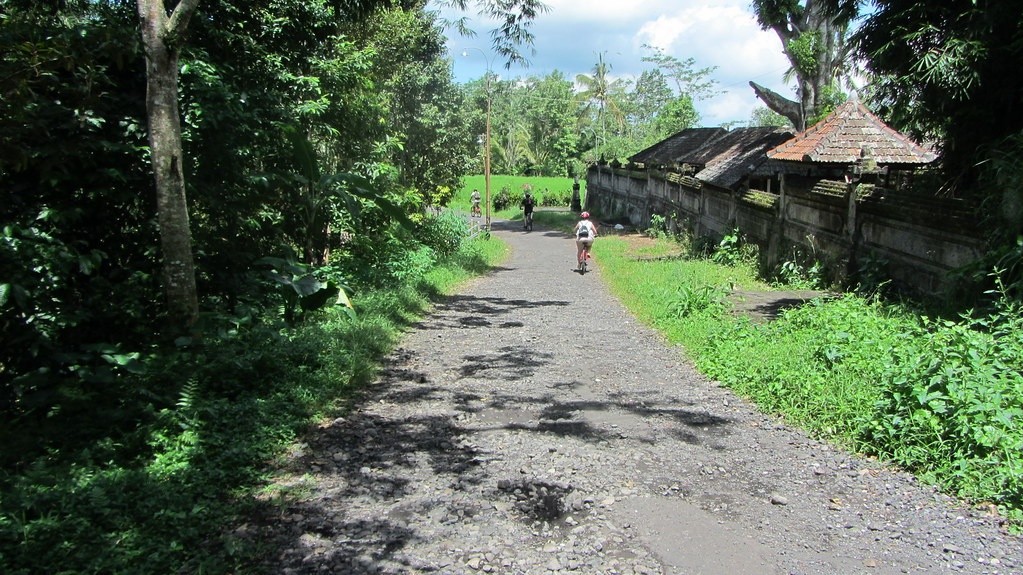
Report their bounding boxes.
[572,234,598,274]
[521,208,533,231]
[470,201,482,218]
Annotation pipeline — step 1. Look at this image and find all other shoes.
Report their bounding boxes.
[587,253,591,258]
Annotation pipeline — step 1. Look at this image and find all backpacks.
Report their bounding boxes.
[474,192,480,199]
[578,221,589,236]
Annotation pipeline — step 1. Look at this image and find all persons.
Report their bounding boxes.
[470,188,481,214]
[520,193,534,229]
[574,212,597,269]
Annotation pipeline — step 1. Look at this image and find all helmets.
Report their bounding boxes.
[580,212,590,219]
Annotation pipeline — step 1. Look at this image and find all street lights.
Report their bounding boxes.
[581,128,597,159]
[460,46,518,230]
